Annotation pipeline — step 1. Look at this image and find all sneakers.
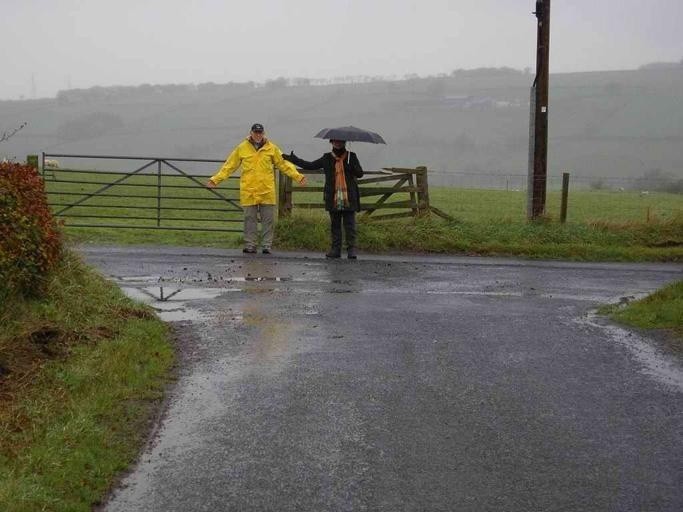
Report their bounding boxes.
[325,251,357,260]
[243,247,272,255]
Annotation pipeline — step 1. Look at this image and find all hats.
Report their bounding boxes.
[252,124,264,132]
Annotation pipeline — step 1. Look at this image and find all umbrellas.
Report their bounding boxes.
[313,123,388,165]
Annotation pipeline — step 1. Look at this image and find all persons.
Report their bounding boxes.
[282,140,366,259]
[205,123,308,255]
